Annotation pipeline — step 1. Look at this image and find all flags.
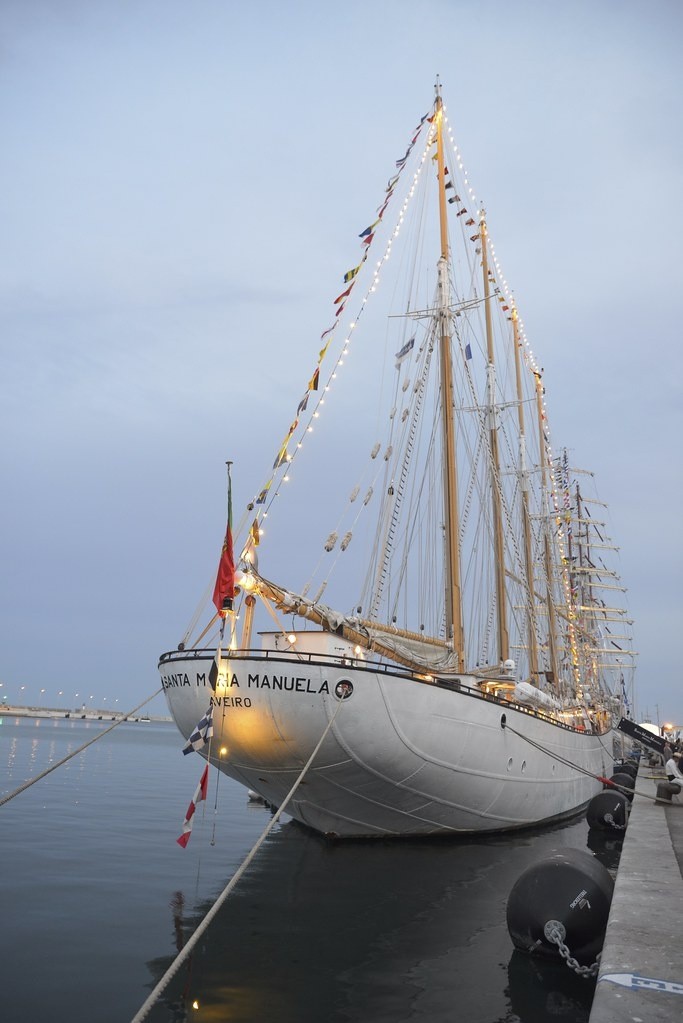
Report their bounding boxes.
[177,764,208,849]
[182,706,213,755]
[536,383,599,730]
[506,317,534,373]
[621,675,630,715]
[248,342,331,546]
[395,338,415,359]
[212,526,235,619]
[488,269,509,312]
[320,113,429,340]
[470,234,484,266]
[446,181,474,226]
[208,640,224,692]
[465,344,472,361]
[427,116,449,179]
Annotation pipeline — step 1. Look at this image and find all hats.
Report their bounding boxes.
[671,752,681,758]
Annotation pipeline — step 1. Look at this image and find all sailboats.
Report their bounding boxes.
[155,74,630,844]
